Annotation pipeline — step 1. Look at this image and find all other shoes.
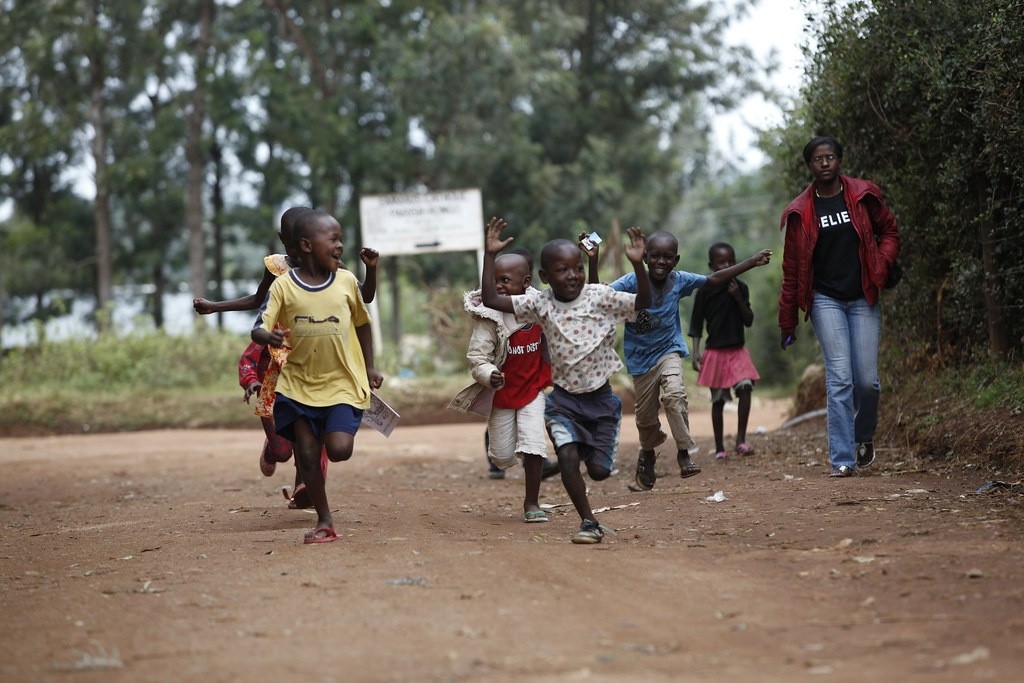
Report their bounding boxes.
[716,451,725,459]
[260,437,276,477]
[489,466,505,479]
[294,483,313,509]
[542,461,561,479]
[289,494,297,509]
[735,444,750,453]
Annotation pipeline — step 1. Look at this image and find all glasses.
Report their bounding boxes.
[810,156,841,165]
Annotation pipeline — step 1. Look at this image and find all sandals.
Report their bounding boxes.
[857,439,876,468]
[829,465,851,477]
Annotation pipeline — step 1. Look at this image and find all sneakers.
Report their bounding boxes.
[572,518,605,544]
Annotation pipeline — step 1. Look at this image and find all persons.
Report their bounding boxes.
[463,254,549,522]
[778,136,901,476]
[238,342,293,477]
[251,210,384,543]
[687,243,761,459]
[481,217,652,544]
[484,250,560,481]
[193,208,379,509]
[578,231,772,490]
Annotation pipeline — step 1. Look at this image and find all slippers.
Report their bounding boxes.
[680,462,701,478]
[636,449,657,491]
[304,527,343,542]
[524,511,548,523]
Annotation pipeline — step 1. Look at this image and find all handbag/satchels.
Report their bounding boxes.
[863,195,903,288]
